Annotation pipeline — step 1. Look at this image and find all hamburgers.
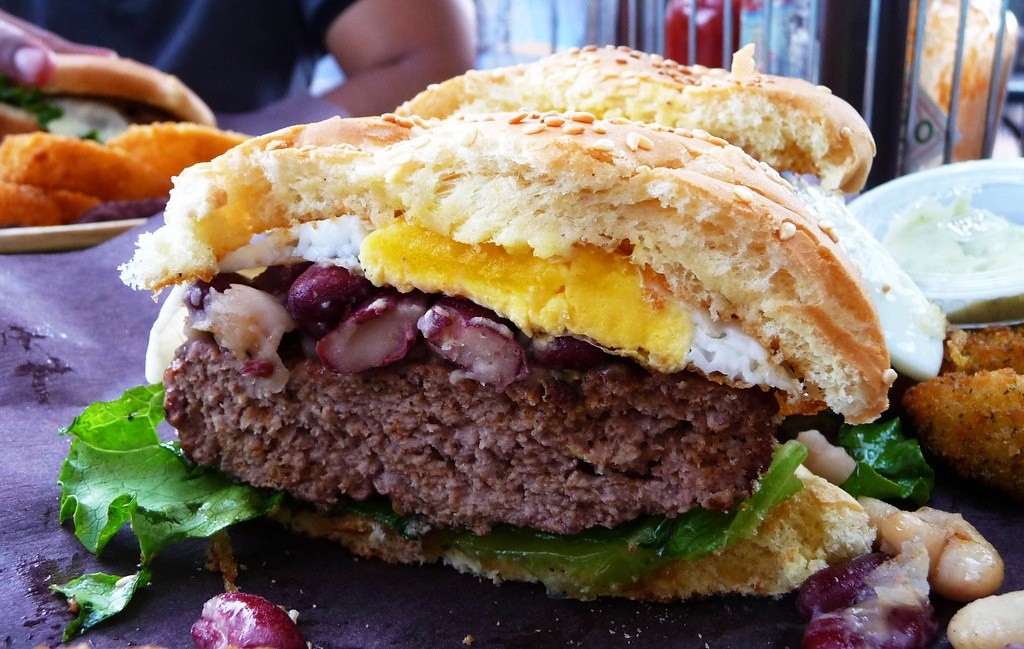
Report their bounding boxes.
[63,108,891,627]
[396,51,870,193]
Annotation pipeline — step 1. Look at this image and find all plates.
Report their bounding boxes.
[0,218,146,252]
[844,158,1024,331]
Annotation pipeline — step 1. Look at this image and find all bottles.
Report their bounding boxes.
[899,0,1018,175]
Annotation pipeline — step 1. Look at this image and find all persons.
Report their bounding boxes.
[0,0,476,152]
